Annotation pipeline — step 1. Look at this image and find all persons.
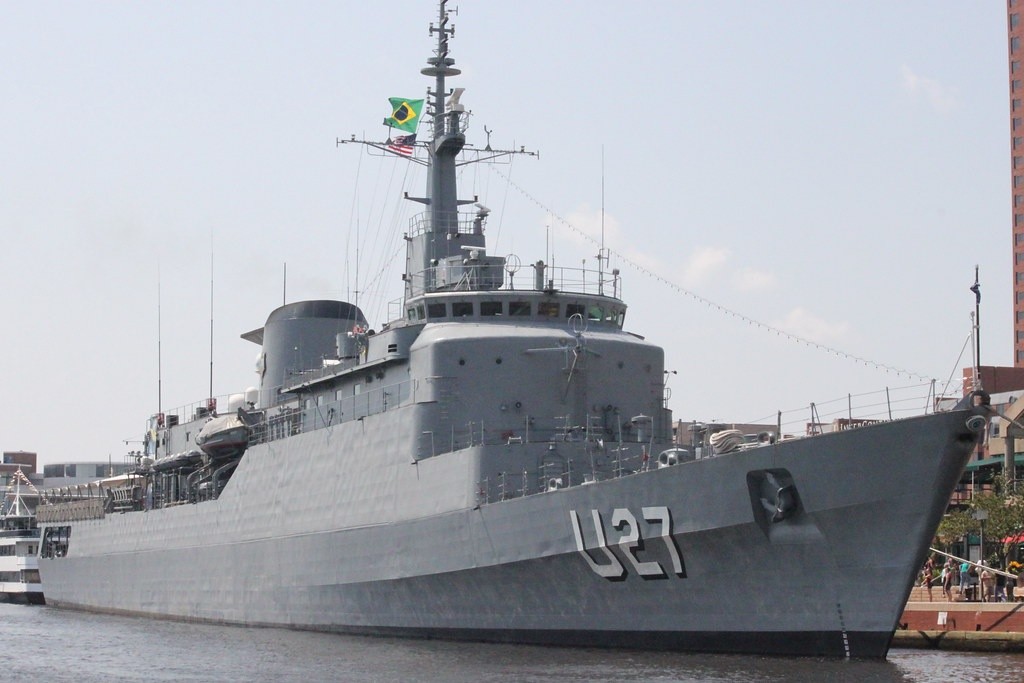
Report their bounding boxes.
[918,555,1007,602]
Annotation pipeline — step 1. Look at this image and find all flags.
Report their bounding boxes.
[390,133,417,159]
[381,97,423,133]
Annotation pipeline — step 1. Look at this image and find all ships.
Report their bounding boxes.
[35,1,990,666]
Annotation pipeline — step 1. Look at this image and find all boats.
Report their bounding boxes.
[0,466,48,603]
